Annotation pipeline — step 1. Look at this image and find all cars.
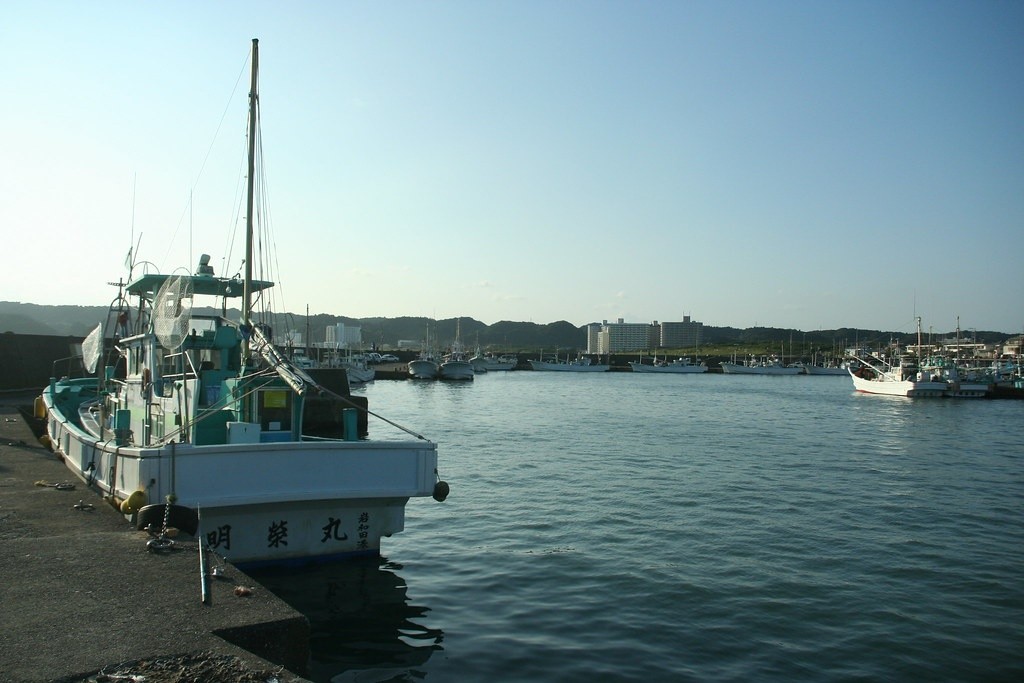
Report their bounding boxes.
[381,354,400,363]
[353,353,382,364]
[787,362,805,368]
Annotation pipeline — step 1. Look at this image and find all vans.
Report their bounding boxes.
[497,354,518,363]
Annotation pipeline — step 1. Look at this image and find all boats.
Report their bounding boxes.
[408,323,439,379]
[33,37,451,579]
[440,319,474,380]
[803,354,849,375]
[628,347,709,373]
[847,353,1024,398]
[282,348,320,370]
[320,356,376,382]
[468,346,488,372]
[718,349,799,374]
[527,348,610,372]
[483,357,514,370]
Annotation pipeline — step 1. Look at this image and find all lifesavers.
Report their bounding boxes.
[133,504,199,535]
[139,367,150,401]
